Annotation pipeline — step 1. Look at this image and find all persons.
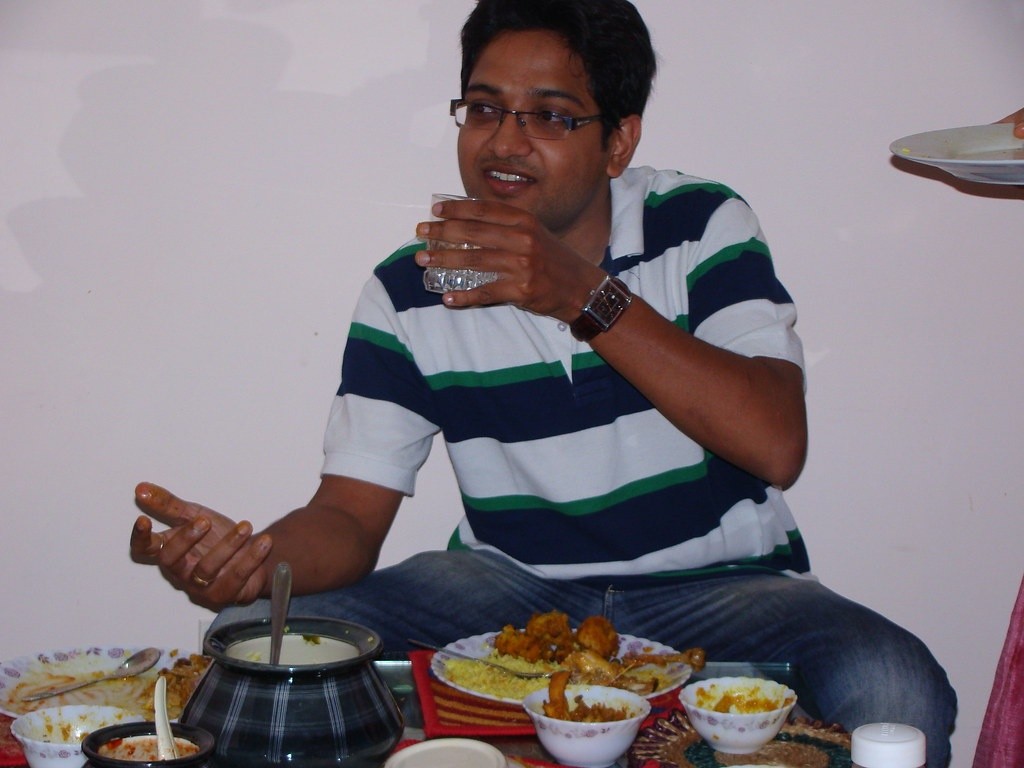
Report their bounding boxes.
[973,107,1024,767]
[130,0,958,768]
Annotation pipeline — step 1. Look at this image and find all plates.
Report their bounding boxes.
[0,642,195,724]
[889,122,1024,186]
[432,629,693,706]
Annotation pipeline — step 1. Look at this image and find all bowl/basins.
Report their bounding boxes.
[79,721,217,768]
[521,684,652,768]
[678,675,798,755]
[9,704,147,768]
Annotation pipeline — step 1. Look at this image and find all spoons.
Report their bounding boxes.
[408,638,553,680]
[21,647,161,701]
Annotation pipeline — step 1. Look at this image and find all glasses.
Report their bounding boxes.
[449,99,608,140]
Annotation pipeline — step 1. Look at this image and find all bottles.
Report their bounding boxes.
[849,722,928,768]
[392,684,425,739]
[179,615,406,768]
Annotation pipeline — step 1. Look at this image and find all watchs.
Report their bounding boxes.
[569,275,632,343]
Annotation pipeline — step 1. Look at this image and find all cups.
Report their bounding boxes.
[380,737,510,768]
[423,192,500,294]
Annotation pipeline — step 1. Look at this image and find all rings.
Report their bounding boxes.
[193,573,213,586]
[159,534,164,550]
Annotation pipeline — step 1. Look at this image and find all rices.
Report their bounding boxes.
[440,646,577,700]
